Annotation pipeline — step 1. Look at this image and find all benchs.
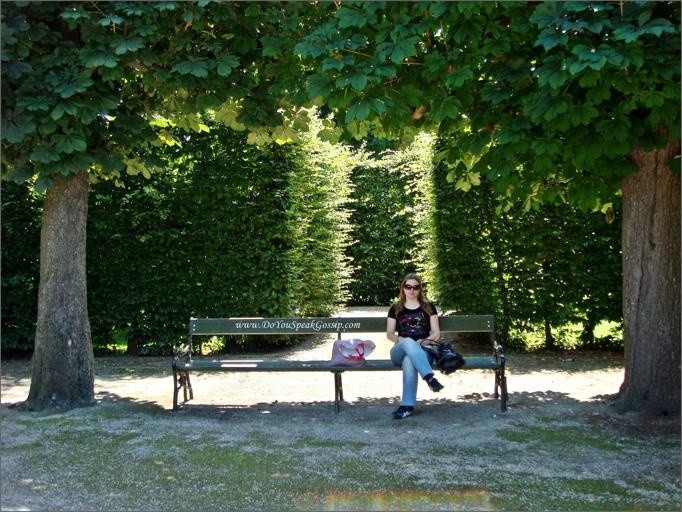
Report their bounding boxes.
[172,315,508,412]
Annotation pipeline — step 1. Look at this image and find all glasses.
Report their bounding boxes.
[404,285,421,290]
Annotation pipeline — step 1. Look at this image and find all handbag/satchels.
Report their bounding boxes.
[331,339,376,368]
[420,339,465,376]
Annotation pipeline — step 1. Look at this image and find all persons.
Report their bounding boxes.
[386,273,444,419]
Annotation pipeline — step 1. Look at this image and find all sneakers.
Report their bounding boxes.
[428,378,444,392]
[392,406,415,419]
